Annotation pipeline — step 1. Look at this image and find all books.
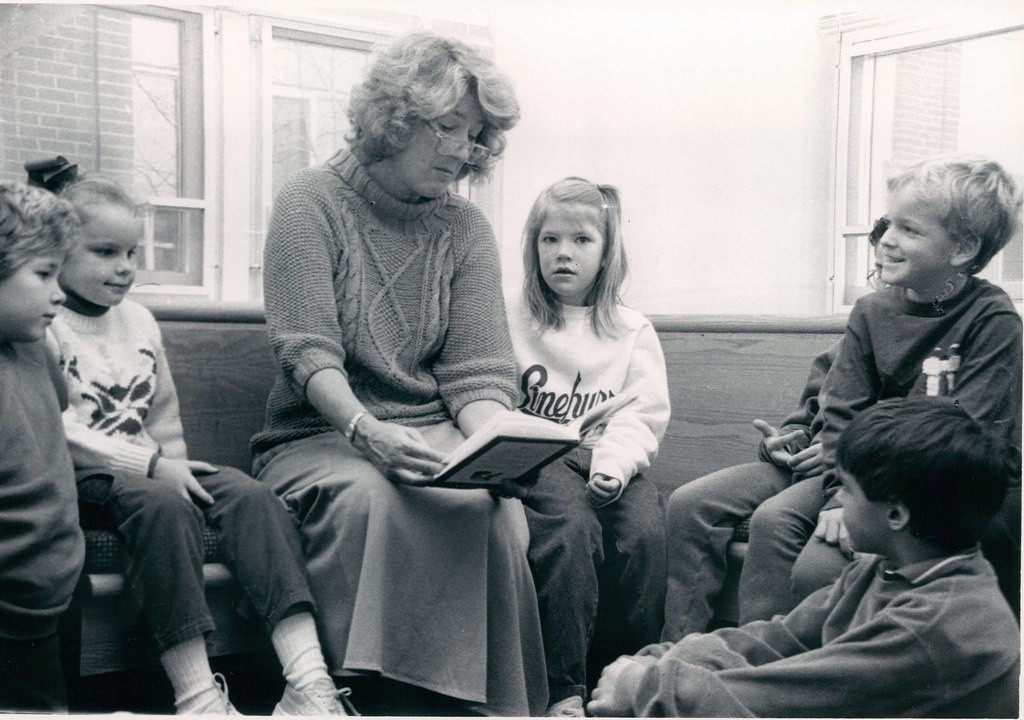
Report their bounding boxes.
[425,394,640,490]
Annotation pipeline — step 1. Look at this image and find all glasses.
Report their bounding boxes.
[422,119,491,166]
[867,267,888,291]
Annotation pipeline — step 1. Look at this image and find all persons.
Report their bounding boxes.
[659,159,1024,648]
[587,394,1020,719]
[44,180,351,716]
[1,176,85,715]
[502,176,671,718]
[251,29,550,718]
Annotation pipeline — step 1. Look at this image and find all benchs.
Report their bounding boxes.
[77,296,850,681]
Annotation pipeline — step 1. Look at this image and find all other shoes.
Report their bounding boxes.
[544,695,585,717]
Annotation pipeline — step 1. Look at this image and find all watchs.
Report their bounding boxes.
[345,409,372,447]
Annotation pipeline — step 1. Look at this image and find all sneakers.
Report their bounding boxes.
[180,673,243,716]
[270,680,360,717]
